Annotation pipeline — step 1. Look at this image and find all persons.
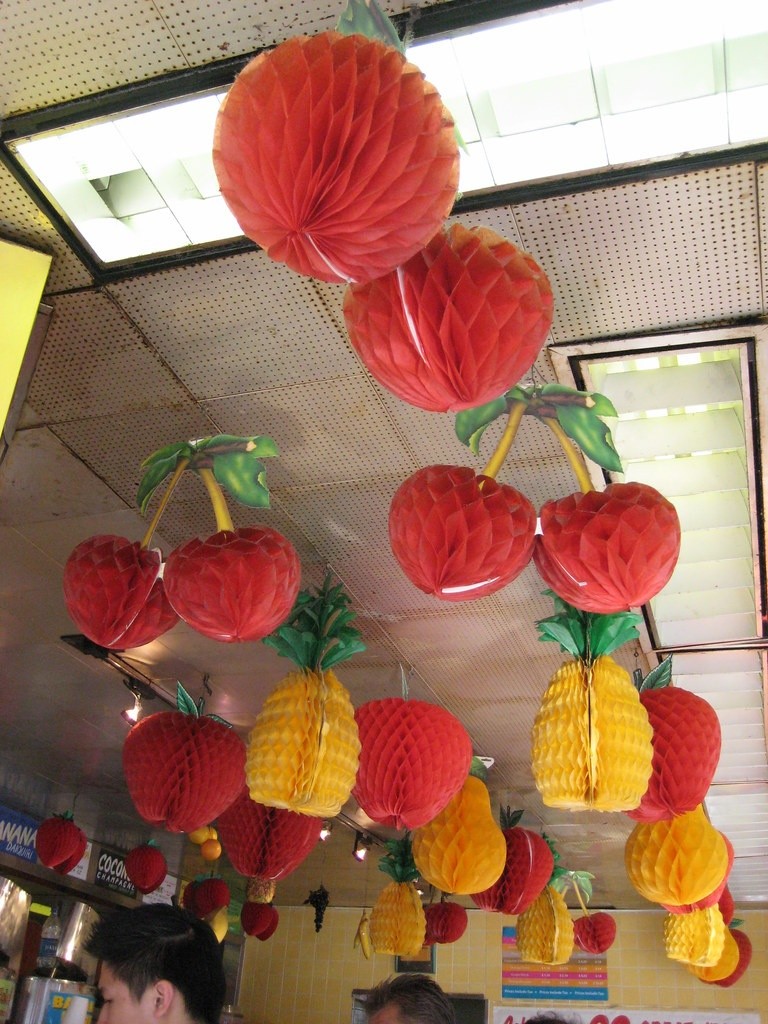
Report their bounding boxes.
[36,957,88,981]
[84,903,225,1024]
[366,975,456,1024]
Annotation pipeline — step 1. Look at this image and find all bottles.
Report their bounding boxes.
[35,905,60,968]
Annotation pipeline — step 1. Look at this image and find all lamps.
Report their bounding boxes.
[351,832,375,863]
[315,821,335,844]
[119,678,158,729]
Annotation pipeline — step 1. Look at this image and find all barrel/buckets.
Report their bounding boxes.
[55,900,103,983]
[12,976,97,1024]
